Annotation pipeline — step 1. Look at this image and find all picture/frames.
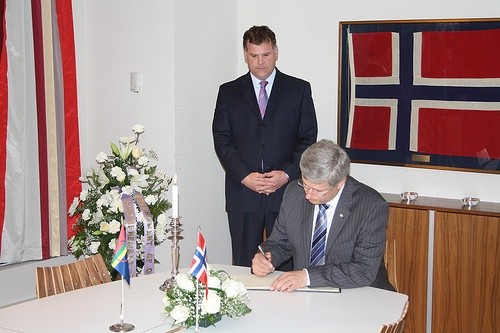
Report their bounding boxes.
[337,19,500,174]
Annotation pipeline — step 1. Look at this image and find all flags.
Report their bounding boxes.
[189,231,209,300]
[112,224,130,285]
[342,24,500,170]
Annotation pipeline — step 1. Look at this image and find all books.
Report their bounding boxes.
[237,273,341,292]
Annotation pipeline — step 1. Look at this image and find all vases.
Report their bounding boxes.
[193,310,221,327]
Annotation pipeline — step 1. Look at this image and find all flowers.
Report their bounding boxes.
[162,270,254,324]
[68,125,182,275]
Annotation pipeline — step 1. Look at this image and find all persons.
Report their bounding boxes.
[212,25,318,272]
[251,139,397,292]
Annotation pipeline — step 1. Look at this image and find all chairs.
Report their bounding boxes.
[35,254,112,301]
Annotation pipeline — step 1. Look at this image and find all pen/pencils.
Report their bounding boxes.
[258,245,274,273]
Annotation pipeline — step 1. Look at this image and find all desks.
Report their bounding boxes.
[0,265,410,333]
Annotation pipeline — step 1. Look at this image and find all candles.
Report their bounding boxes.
[172,175,179,217]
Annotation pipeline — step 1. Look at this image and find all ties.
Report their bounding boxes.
[309,203,331,267]
[257,80,269,119]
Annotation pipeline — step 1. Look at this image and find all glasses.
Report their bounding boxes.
[297,176,330,197]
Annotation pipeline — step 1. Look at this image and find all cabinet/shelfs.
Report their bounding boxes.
[263,194,500,333]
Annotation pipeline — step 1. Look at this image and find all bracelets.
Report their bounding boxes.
[286,174,291,182]
